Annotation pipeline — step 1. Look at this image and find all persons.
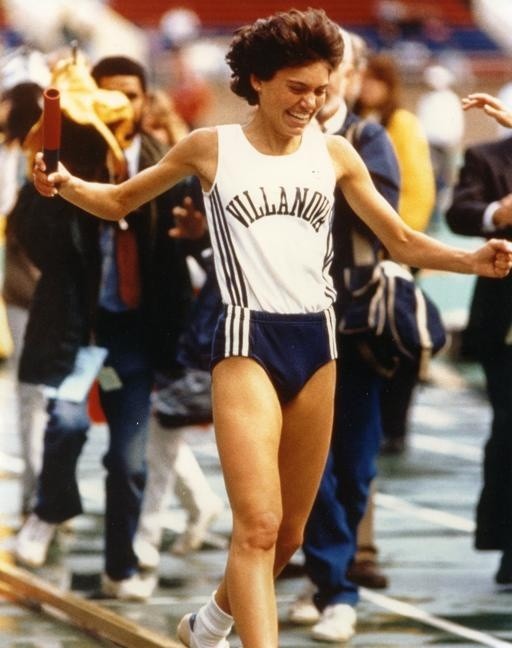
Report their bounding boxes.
[1,9,512,648]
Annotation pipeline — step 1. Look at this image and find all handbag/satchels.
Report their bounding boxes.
[335,261,446,378]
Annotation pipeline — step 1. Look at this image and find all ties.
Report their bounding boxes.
[112,144,142,311]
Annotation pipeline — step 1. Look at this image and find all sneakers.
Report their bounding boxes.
[177,613,231,648]
[12,494,74,568]
[345,558,387,590]
[173,496,228,554]
[311,601,359,643]
[286,584,319,626]
[101,563,161,601]
